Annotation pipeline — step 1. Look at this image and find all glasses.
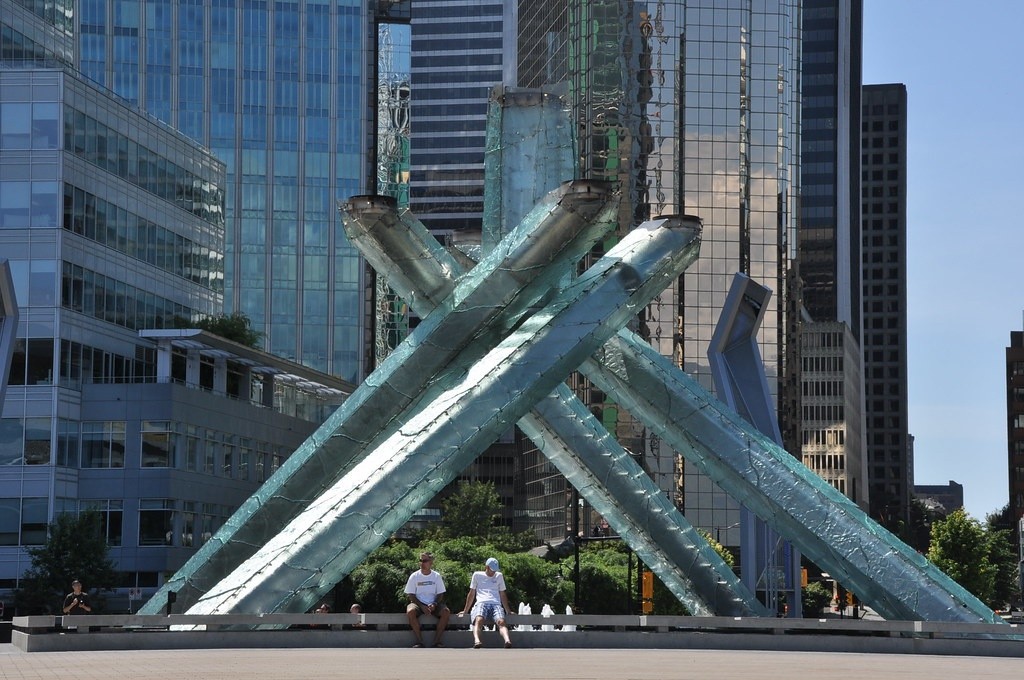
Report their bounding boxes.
[419,559,430,563]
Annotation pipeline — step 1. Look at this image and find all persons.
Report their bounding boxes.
[404,552,450,648]
[350,604,365,627]
[459,557,515,648]
[321,604,331,613]
[63,580,92,615]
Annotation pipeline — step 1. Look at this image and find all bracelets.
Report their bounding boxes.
[433,602,437,606]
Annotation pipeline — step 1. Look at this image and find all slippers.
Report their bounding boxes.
[432,642,446,647]
[412,643,424,648]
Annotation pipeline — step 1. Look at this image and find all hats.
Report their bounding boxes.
[486,558,498,572]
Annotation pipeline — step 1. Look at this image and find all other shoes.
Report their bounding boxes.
[504,641,511,648]
[475,642,482,648]
